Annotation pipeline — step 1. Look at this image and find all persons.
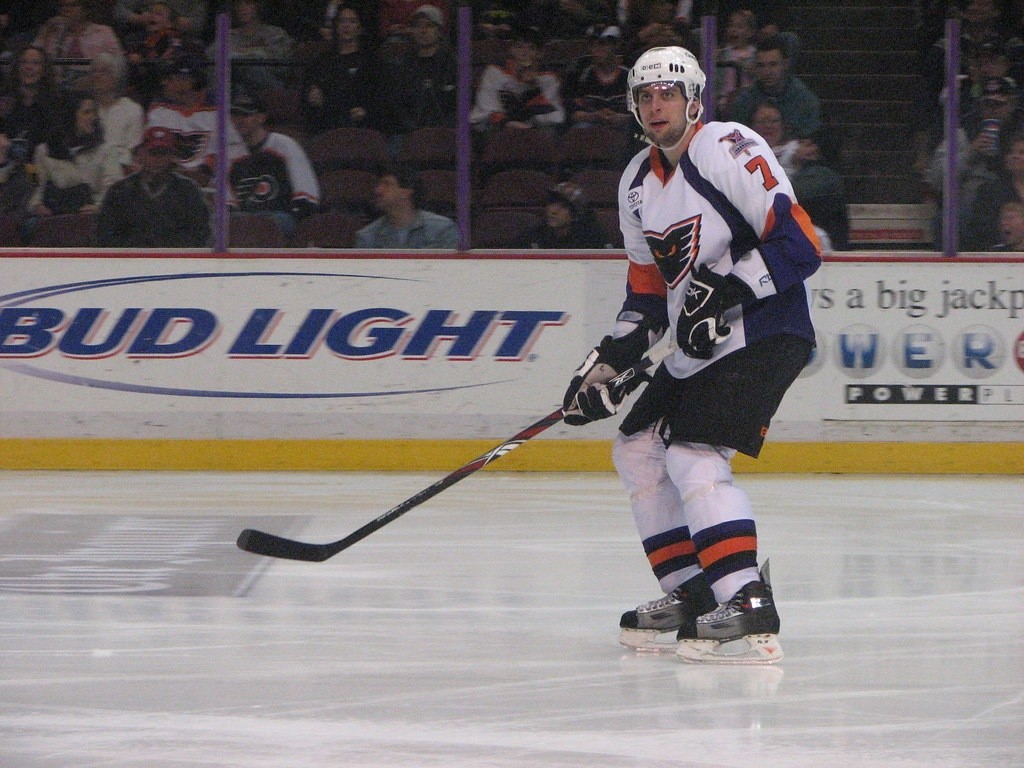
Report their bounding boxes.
[386,4,477,133]
[0,0,386,163]
[928,0,1024,251]
[727,41,821,140]
[562,45,821,639]
[28,92,124,216]
[566,0,777,123]
[213,95,320,215]
[516,173,617,249]
[354,163,458,249]
[469,27,565,130]
[98,126,211,247]
[793,131,850,251]
[751,103,821,186]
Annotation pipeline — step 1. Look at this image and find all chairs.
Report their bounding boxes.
[0,0,730,248]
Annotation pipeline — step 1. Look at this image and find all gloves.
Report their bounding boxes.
[675,263,734,360]
[560,334,631,426]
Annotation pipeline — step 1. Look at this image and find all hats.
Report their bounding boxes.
[598,26,623,40]
[140,124,175,147]
[979,76,1020,102]
[511,25,547,42]
[407,4,444,26]
[228,91,270,115]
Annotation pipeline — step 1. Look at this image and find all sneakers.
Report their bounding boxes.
[672,580,782,663]
[618,592,684,652]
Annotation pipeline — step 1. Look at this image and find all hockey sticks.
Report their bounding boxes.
[234,318,725,562]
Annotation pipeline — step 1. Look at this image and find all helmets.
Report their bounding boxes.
[626,45,707,111]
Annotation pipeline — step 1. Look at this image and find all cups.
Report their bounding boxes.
[981,119,1000,154]
